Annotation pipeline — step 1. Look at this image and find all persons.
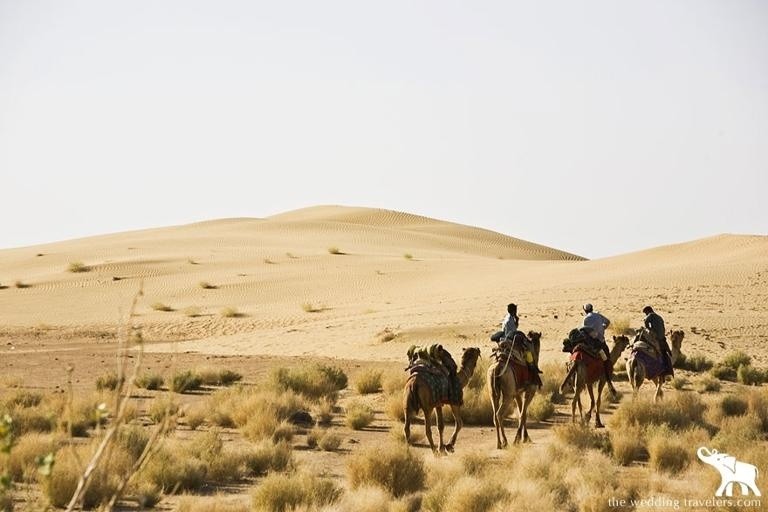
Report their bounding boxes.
[643,306,666,341]
[583,304,610,358]
[502,303,543,373]
